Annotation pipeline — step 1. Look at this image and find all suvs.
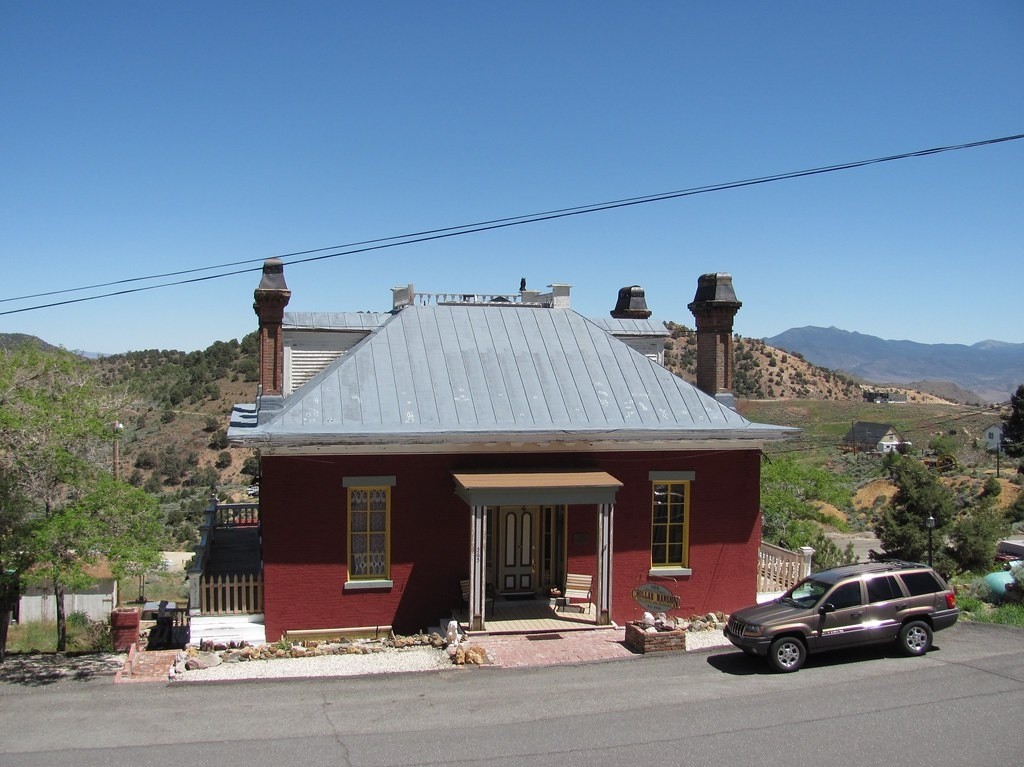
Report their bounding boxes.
[723,561,958,671]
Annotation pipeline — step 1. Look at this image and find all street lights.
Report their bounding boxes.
[926,516,935,567]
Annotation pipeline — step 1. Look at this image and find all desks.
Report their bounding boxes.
[142,601,178,620]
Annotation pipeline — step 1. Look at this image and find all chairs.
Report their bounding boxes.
[562,573,593,614]
[459,579,495,617]
